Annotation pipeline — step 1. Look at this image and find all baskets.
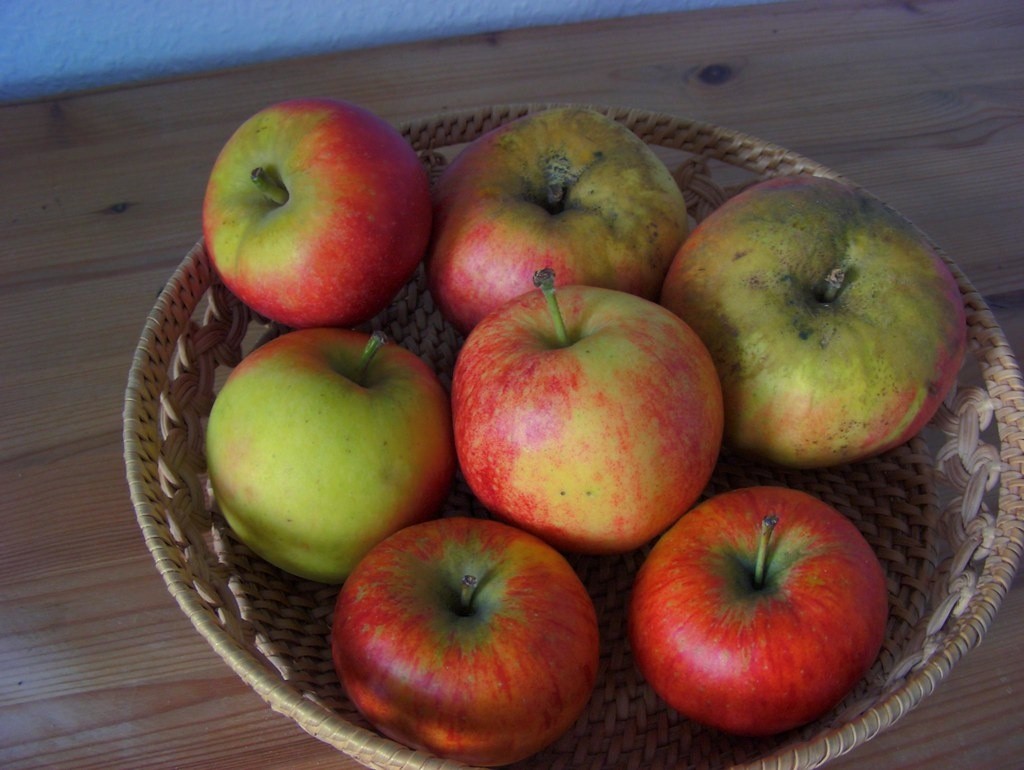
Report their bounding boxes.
[123,102,1024,769]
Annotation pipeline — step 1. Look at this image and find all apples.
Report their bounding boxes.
[330,513,598,767]
[449,266,727,557]
[625,485,891,739]
[199,97,432,331]
[424,107,687,335]
[204,326,455,587]
[659,173,968,471]
